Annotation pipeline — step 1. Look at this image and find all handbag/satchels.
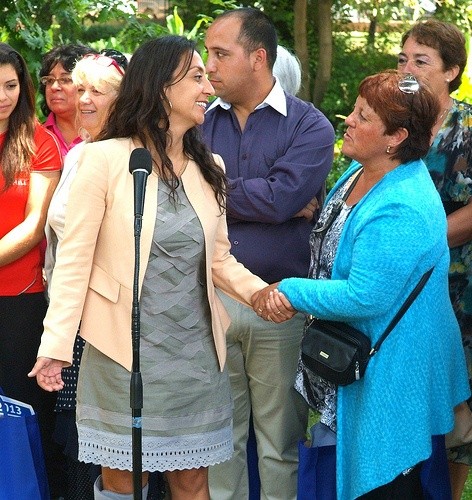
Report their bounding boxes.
[300,318,371,386]
[0,388,51,500]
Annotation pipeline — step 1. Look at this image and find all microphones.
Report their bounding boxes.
[129,148,152,236]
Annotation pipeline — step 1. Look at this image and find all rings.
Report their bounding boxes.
[276,311,280,316]
[258,308,264,313]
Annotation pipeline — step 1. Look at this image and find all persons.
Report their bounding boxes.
[39,43,91,156]
[191,9,335,500]
[251,70,472,500]
[55,49,133,500]
[27,35,293,499]
[396,16,472,499]
[273,41,326,221]
[0,40,62,500]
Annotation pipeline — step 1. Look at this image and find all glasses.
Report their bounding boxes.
[83,54,125,77]
[39,76,73,86]
[382,68,419,129]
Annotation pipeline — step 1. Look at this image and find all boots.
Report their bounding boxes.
[94,475,149,500]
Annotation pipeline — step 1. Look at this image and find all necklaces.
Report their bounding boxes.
[177,158,187,176]
